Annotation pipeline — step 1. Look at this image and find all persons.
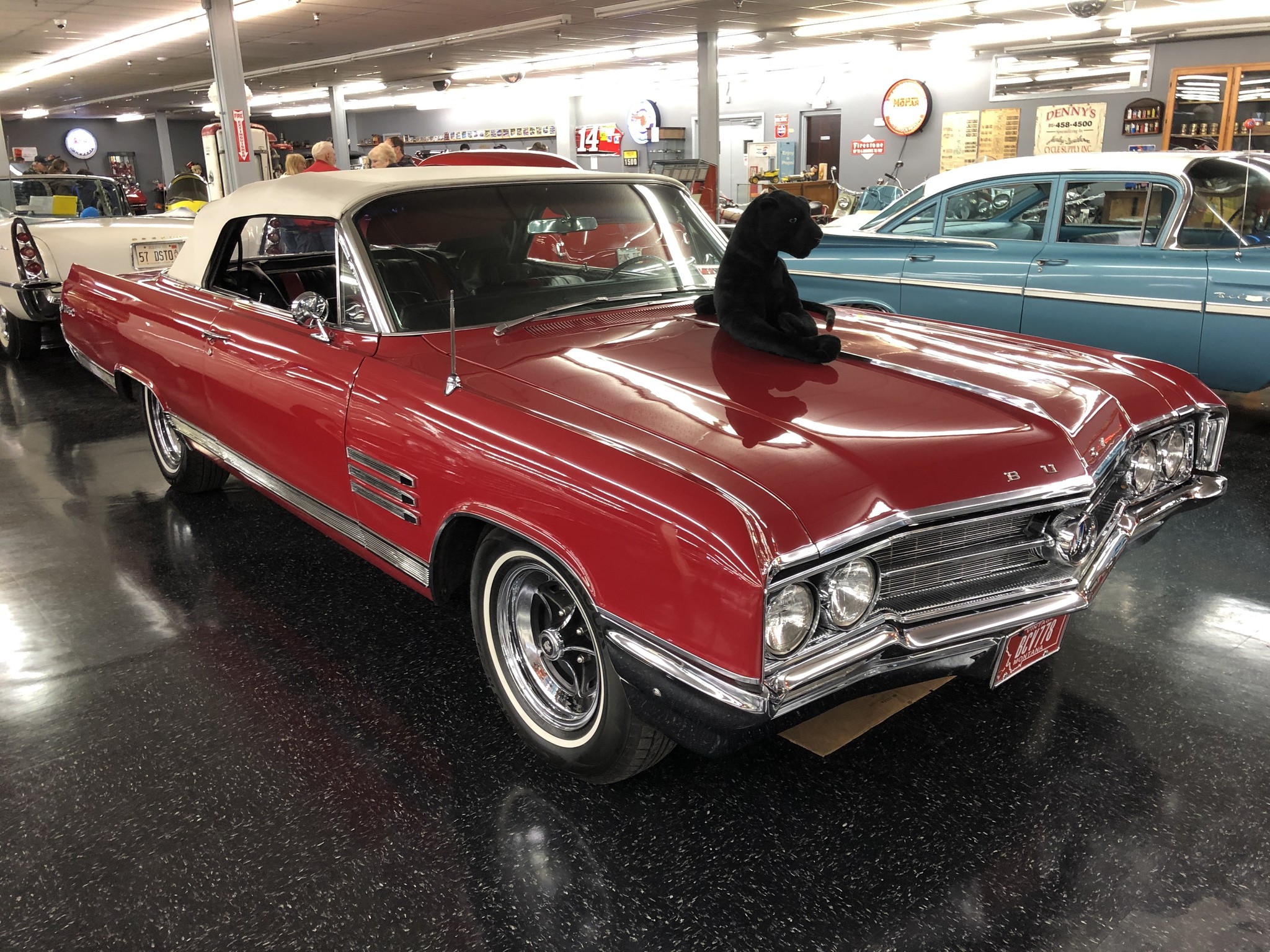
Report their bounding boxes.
[362,136,418,247]
[494,144,507,149]
[187,161,206,181]
[10,155,111,217]
[528,141,549,152]
[279,140,342,255]
[461,144,470,150]
[1202,175,1243,229]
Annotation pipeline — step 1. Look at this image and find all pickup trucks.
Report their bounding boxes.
[0,174,267,363]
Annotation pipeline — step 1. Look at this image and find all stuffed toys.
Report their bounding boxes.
[711,331,839,448]
[694,190,841,363]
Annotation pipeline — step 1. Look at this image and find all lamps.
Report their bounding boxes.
[0,0,1270,118]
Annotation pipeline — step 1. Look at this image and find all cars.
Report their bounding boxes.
[97,175,147,216]
[358,149,692,271]
[777,151,1270,412]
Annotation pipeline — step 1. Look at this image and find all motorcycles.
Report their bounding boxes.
[153,171,214,213]
[60,166,1229,785]
[946,184,1106,224]
[700,161,911,225]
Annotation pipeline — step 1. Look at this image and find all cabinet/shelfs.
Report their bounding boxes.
[1122,97,1165,135]
[1163,62,1270,152]
[760,180,837,215]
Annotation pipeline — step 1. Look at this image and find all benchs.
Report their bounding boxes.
[1070,230,1152,246]
[237,248,540,317]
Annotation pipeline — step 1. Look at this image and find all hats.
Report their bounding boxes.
[494,144,507,149]
[528,141,546,151]
[460,144,470,150]
[34,155,47,163]
